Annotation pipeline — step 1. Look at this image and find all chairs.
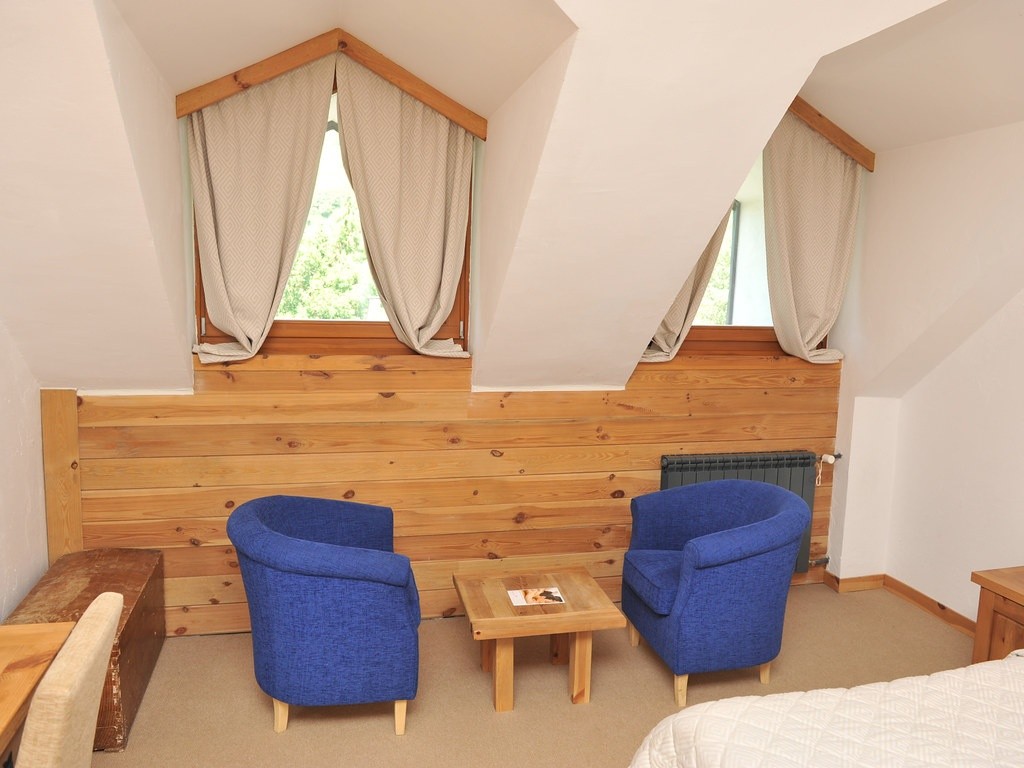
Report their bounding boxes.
[11,591,124,768]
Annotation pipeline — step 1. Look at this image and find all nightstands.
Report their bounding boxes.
[970,563,1023,665]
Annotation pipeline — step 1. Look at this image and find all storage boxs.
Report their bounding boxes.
[4,547,167,754]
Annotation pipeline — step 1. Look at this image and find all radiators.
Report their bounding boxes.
[659,448,818,578]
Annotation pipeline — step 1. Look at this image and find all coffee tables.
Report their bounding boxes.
[449,564,630,713]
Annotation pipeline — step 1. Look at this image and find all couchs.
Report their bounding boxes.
[223,495,422,736]
[621,479,813,710]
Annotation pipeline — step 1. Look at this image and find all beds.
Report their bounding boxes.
[626,649,1024,768]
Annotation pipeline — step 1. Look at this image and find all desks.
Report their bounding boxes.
[1,619,75,757]
